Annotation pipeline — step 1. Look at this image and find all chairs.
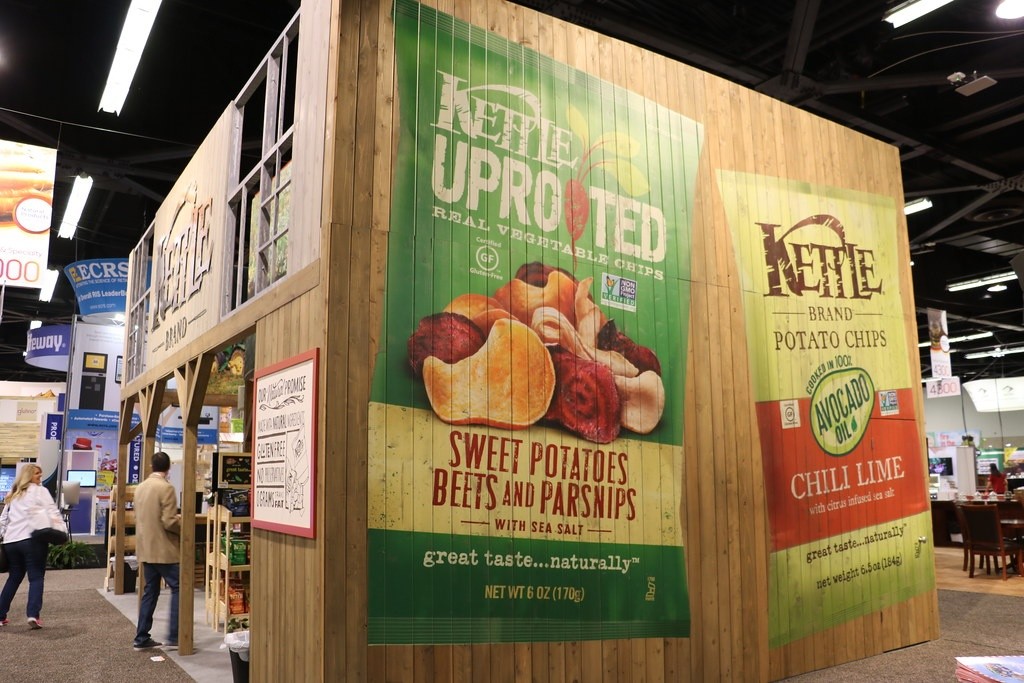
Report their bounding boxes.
[953,501,1024,581]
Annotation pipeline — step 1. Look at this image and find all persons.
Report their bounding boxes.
[0,464,70,629]
[1013,465,1024,478]
[133,452,182,652]
[930,462,944,475]
[985,463,1008,495]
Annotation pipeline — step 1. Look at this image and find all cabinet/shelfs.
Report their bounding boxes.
[204,491,251,636]
[103,485,136,593]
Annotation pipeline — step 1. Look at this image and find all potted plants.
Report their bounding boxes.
[48,541,101,570]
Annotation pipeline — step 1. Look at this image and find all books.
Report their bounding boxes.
[954,654,1024,683]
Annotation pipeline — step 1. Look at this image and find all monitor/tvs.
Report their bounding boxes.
[929,457,953,476]
[67,470,96,488]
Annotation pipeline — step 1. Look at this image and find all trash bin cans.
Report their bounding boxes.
[110,555,138,593]
[223,630,250,683]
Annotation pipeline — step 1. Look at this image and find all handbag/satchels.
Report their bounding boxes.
[31,510,69,544]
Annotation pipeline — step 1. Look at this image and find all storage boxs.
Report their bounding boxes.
[232,540,250,565]
[224,578,251,614]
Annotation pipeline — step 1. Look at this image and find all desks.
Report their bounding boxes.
[931,494,1024,546]
[162,514,226,590]
[1001,519,1024,540]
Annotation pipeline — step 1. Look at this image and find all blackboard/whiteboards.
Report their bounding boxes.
[217,451,251,489]
[251,347,320,539]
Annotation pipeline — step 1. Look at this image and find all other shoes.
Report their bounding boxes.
[165,642,178,649]
[0,619,9,626]
[27,617,42,628]
[134,639,162,651]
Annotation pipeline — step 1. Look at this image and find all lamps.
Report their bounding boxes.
[57,171,93,240]
[903,197,933,216]
[97,0,163,117]
[882,0,955,29]
[945,271,1018,292]
